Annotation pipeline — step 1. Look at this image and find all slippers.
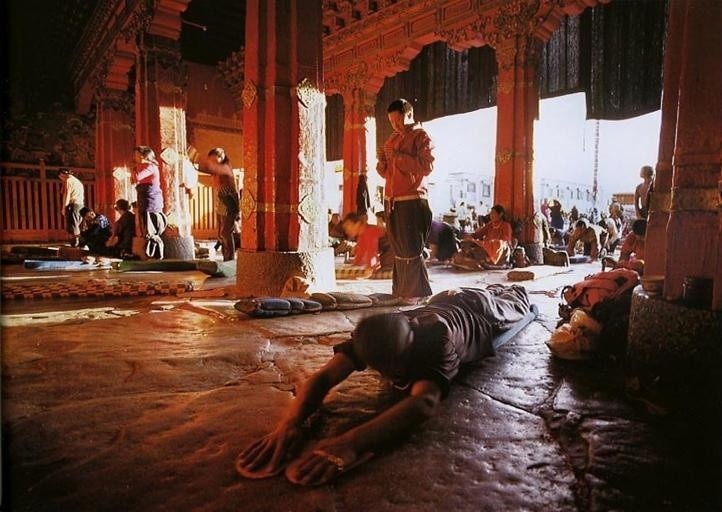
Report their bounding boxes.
[233,438,378,489]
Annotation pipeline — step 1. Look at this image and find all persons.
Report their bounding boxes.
[130,202,137,217]
[539,196,637,261]
[189,147,240,262]
[376,100,436,304]
[57,168,84,248]
[76,207,113,251]
[214,188,242,254]
[106,199,134,257]
[130,146,168,261]
[327,209,395,273]
[421,201,512,270]
[238,284,530,484]
[597,166,652,275]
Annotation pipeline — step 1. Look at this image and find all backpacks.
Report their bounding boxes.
[561,268,637,312]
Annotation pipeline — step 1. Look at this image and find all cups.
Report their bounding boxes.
[640,274,664,296]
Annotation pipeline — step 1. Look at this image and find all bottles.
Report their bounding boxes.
[516,247,526,268]
[345,251,350,262]
[681,276,704,307]
